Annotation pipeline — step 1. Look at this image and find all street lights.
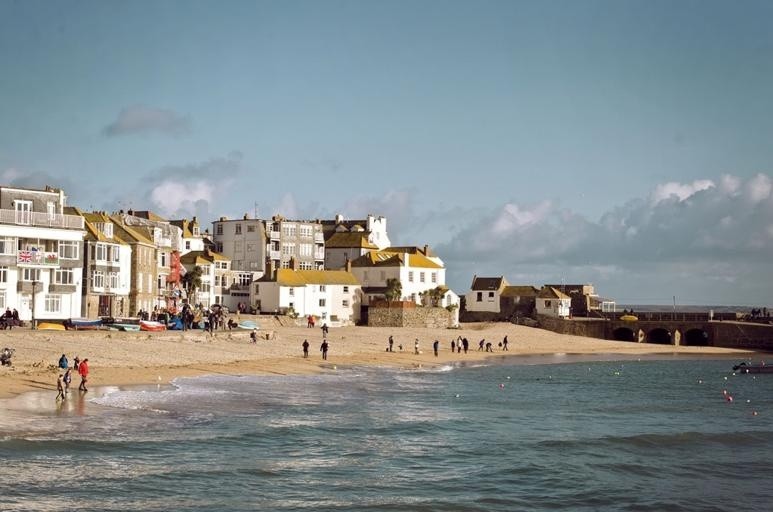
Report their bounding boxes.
[30,280,36,329]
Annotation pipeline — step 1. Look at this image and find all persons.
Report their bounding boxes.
[477,338,484,352]
[5,306,13,320]
[77,358,89,392]
[55,374,64,402]
[461,338,469,354]
[76,391,87,417]
[58,354,68,369]
[12,308,19,319]
[301,338,309,359]
[0,313,8,330]
[62,368,71,393]
[249,329,257,343]
[456,336,463,354]
[73,356,79,371]
[413,339,419,355]
[319,339,328,361]
[501,335,509,352]
[485,342,492,352]
[388,335,393,352]
[306,314,313,329]
[136,301,262,332]
[450,338,455,353]
[0,347,11,366]
[432,340,439,357]
[319,323,328,339]
[53,400,64,417]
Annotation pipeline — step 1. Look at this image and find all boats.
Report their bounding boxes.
[35,316,258,333]
[739,362,773,375]
